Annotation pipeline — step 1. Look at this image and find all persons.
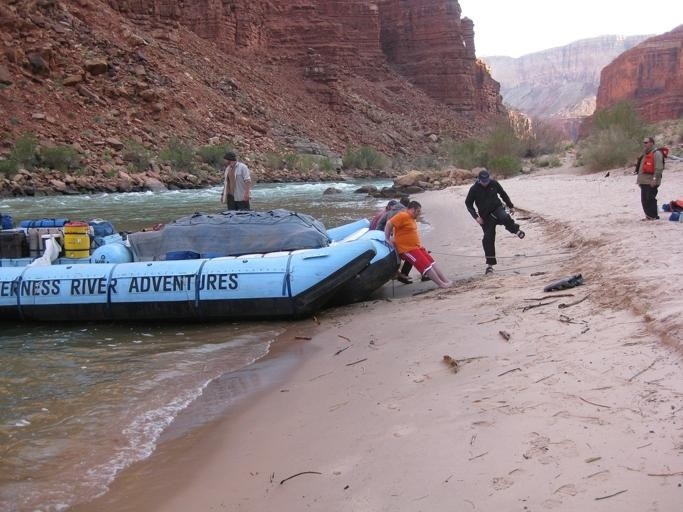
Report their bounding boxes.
[374,196,432,285]
[218,152,253,212]
[383,201,457,289]
[464,169,526,275]
[631,135,669,222]
[368,198,397,231]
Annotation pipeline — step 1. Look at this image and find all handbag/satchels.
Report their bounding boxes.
[642,152,653,174]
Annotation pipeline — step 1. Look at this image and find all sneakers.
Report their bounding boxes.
[397,273,412,284]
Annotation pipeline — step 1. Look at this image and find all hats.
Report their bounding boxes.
[224,152,237,161]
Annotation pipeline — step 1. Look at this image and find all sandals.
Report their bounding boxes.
[486,267,493,274]
[515,229,525,239]
